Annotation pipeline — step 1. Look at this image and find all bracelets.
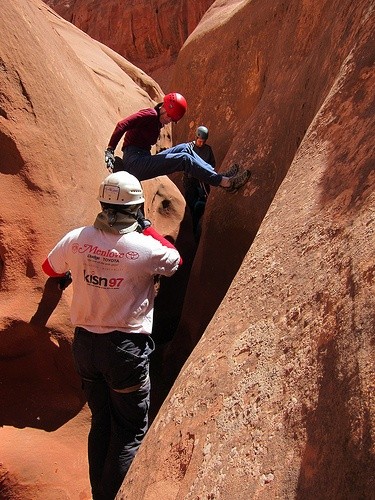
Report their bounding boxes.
[107,148,112,151]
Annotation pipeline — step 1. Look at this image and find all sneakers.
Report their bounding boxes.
[222,169,251,194]
[218,163,239,188]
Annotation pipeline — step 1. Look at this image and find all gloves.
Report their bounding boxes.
[105,148,115,169]
[58,270,73,291]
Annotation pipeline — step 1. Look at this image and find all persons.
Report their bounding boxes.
[156,125,216,244]
[104,92,252,195]
[43,171,184,500]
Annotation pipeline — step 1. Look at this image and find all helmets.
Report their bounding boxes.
[163,93,187,121]
[196,126,209,140]
[96,171,146,205]
